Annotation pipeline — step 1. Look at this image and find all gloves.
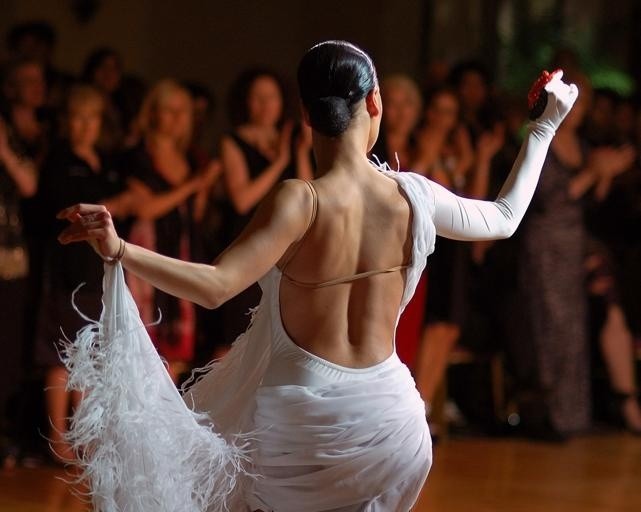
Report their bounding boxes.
[528,69,579,136]
